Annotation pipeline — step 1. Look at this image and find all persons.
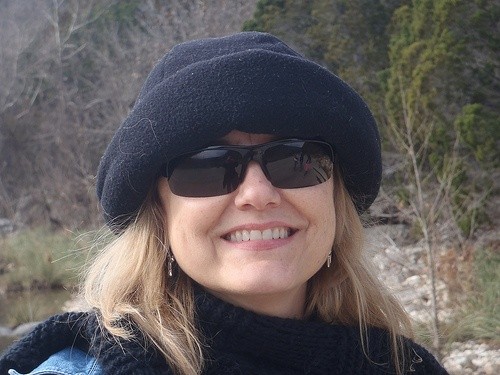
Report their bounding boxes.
[293,150,314,176]
[0,32,448,375]
[217,149,244,194]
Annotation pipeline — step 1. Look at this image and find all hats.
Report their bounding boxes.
[95,31,385,236]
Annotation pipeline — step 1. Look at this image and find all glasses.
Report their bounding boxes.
[159,135,336,198]
